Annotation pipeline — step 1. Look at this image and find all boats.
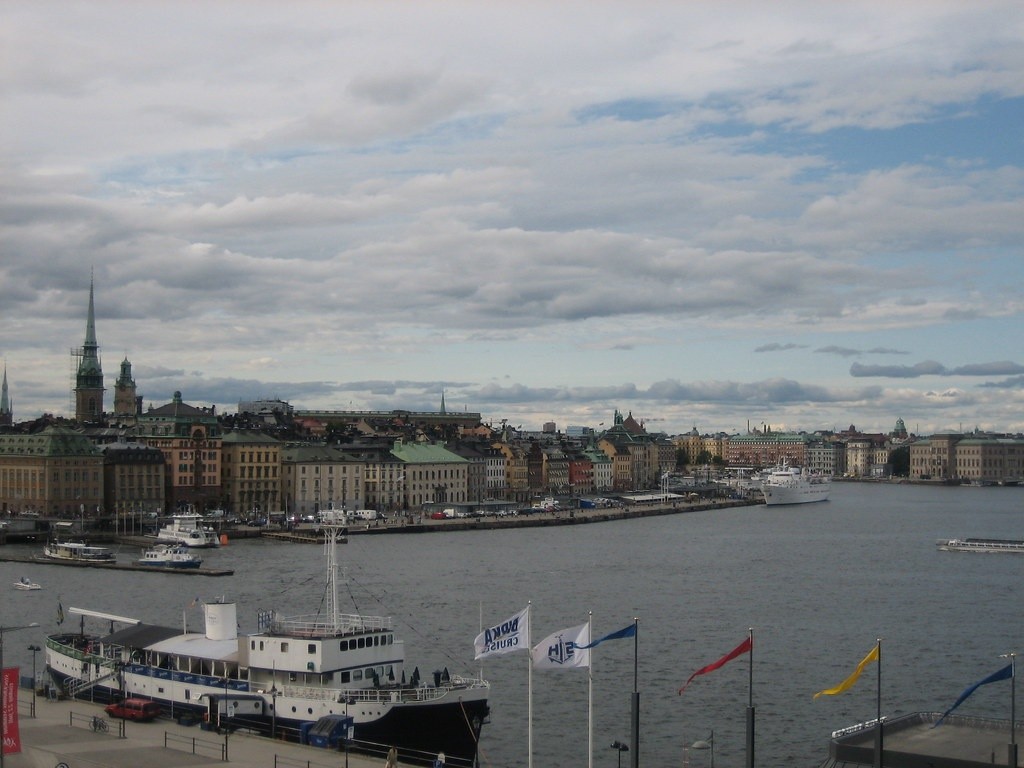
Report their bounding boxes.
[157,503,224,548]
[42,535,119,564]
[750,454,832,506]
[44,499,493,768]
[12,577,43,591]
[137,539,205,570]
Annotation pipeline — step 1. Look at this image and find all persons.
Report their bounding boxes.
[387,745,398,768]
[436,752,445,768]
[21,575,31,585]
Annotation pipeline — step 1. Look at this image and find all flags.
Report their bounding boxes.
[57,601,64,626]
[532,622,589,672]
[474,606,528,660]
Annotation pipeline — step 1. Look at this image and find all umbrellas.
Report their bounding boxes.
[373,669,380,690]
[442,667,450,681]
[410,666,420,688]
[388,667,395,681]
[402,670,405,684]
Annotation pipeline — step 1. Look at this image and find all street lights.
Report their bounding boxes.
[0,622,43,768]
[116,660,132,738]
[336,698,358,768]
[690,730,715,768]
[218,677,233,760]
[27,644,42,718]
[610,740,630,768]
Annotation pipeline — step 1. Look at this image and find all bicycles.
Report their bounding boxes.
[88,716,110,733]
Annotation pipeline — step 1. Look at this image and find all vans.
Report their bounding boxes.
[104,699,161,722]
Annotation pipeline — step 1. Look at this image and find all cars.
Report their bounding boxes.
[228,506,386,528]
[431,504,568,521]
